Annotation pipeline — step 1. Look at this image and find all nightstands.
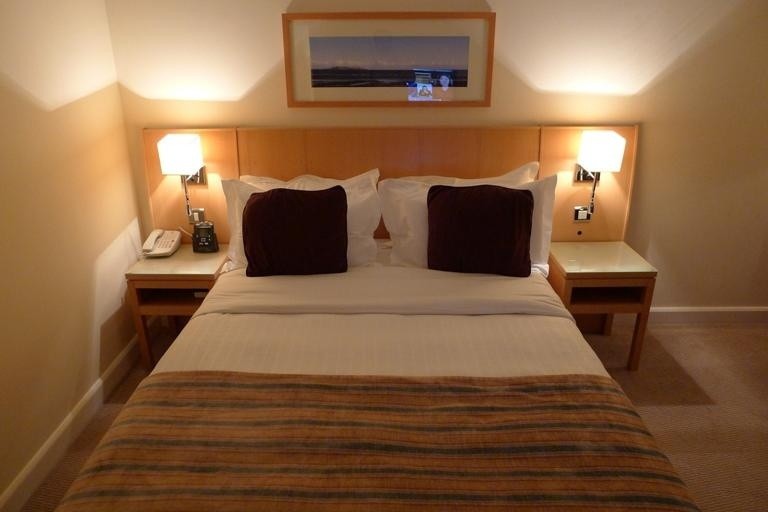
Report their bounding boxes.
[122,243,225,368]
[546,240,658,374]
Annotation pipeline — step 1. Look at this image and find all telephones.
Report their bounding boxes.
[142,229,181,257]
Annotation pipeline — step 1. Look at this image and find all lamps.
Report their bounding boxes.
[569,129,627,183]
[156,133,206,185]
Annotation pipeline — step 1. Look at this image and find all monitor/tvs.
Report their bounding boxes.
[406,66,454,103]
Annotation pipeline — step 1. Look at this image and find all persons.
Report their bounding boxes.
[418,85,431,97]
[431,73,453,99]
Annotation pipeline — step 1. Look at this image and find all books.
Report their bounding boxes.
[194,290,208,299]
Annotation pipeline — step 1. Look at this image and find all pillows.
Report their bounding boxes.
[376,172,557,277]
[426,181,533,276]
[237,167,379,190]
[242,185,350,277]
[221,177,379,273]
[394,161,540,182]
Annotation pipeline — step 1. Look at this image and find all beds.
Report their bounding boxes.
[52,115,700,510]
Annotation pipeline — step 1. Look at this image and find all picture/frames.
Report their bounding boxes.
[281,10,497,109]
